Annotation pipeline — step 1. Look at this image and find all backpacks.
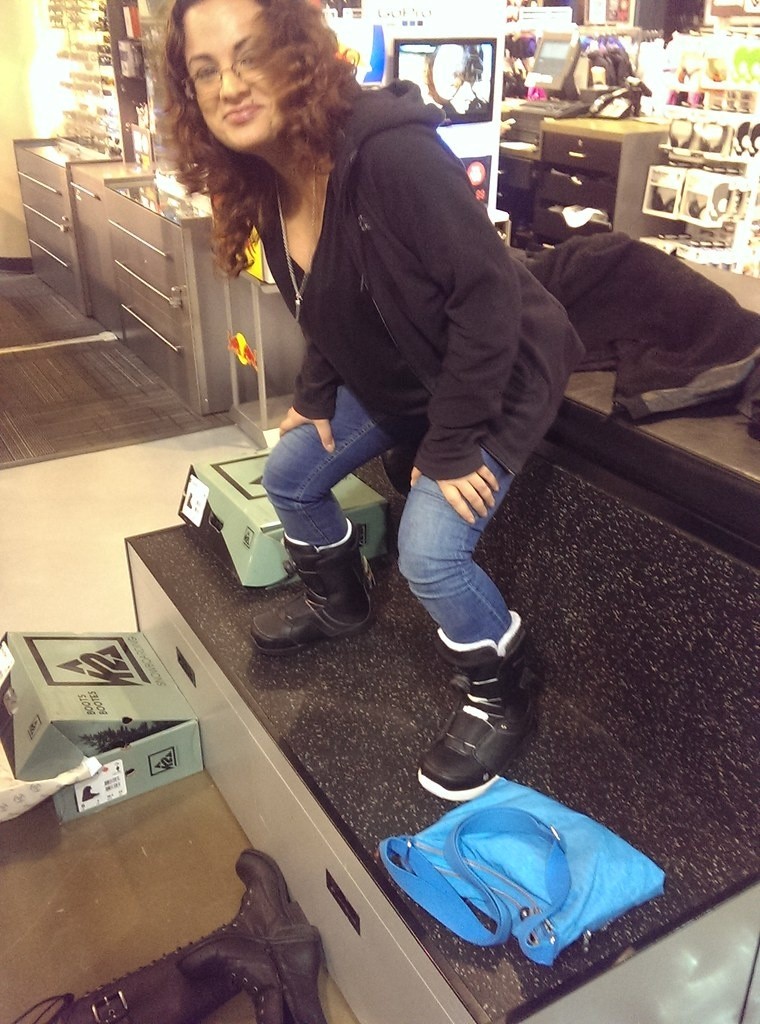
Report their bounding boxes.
[572,37,652,117]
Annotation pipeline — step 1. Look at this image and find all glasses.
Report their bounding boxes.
[182,51,271,101]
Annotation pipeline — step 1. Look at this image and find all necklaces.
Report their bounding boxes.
[278,148,318,321]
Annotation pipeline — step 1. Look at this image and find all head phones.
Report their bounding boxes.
[650,118,760,221]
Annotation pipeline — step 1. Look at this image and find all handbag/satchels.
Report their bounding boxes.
[379,776,666,964]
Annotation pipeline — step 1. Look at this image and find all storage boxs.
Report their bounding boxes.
[0,629,207,827]
[177,443,392,592]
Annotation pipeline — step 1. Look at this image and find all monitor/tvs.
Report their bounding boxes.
[524,31,580,90]
[393,38,496,124]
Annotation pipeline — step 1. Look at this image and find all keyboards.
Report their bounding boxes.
[521,100,568,110]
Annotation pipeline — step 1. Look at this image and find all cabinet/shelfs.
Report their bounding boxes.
[14,137,122,317]
[533,117,679,252]
[124,437,760,1024]
[67,160,240,416]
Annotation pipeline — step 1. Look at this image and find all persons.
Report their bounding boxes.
[156,0,585,804]
[425,38,491,121]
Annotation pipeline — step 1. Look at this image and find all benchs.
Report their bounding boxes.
[517,245,760,549]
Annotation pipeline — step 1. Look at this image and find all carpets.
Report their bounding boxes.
[0,272,245,470]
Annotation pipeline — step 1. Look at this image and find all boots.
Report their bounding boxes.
[418,610,540,801]
[175,924,326,1024]
[13,848,309,1024]
[251,517,375,656]
